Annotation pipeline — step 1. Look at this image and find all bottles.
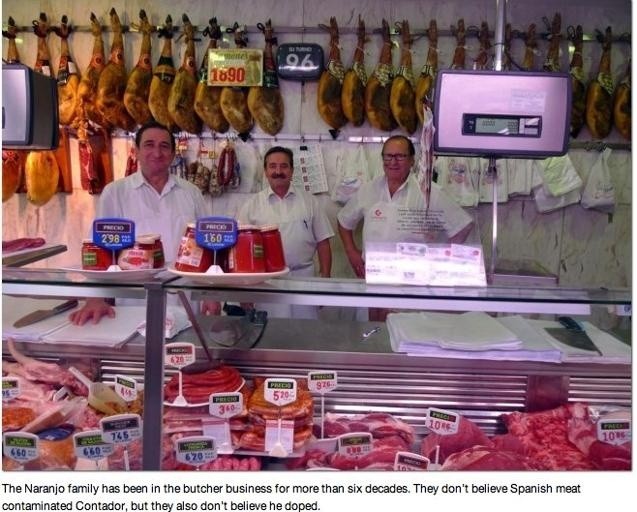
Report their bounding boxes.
[174,222,286,272]
[81,234,164,271]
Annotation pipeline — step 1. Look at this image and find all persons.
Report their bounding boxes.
[67,118,222,329]
[336,136,474,322]
[225,143,337,318]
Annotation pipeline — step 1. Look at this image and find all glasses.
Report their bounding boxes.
[383,149,408,161]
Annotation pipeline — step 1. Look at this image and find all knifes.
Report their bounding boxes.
[545,316,603,354]
[14,299,79,331]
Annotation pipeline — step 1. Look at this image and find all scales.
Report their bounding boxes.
[430,70,572,286]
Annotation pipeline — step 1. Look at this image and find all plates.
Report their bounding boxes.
[154,379,248,410]
[167,263,284,286]
[62,267,168,281]
